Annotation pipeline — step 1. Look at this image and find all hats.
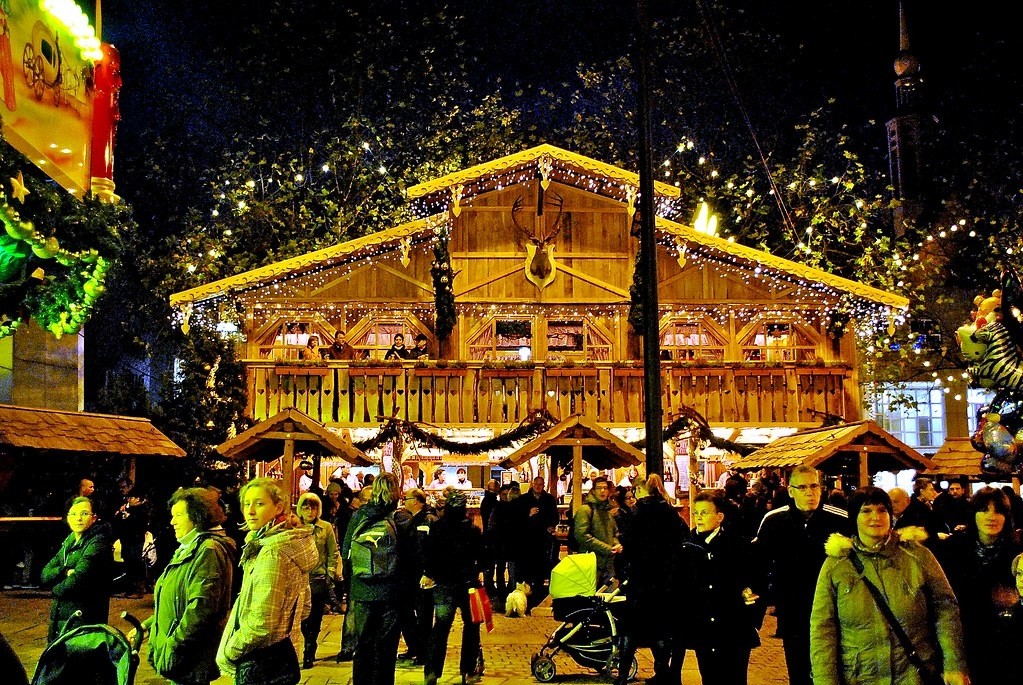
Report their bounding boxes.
[124,487,144,498]
[327,482,342,494]
[413,334,428,341]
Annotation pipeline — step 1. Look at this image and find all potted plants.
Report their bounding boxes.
[482,356,533,377]
[545,358,597,377]
[671,358,727,375]
[611,359,664,376]
[348,357,402,376]
[413,357,469,377]
[794,356,850,377]
[275,356,329,377]
[732,360,789,376]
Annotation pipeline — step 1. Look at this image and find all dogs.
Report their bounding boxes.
[504,582,532,618]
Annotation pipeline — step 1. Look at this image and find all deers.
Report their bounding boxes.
[511,189,564,279]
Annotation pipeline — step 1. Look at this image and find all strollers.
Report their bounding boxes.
[530,552,638,683]
[30,610,144,685]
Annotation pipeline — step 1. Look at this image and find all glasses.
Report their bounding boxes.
[790,483,821,491]
[532,482,544,487]
[691,508,717,517]
[300,505,319,511]
[624,496,635,500]
[404,497,416,501]
[67,509,95,519]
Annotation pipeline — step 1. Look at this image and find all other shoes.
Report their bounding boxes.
[302,659,313,669]
[413,658,427,665]
[336,649,356,662]
[398,652,413,660]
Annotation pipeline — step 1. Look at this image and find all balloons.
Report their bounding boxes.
[955,269,1023,477]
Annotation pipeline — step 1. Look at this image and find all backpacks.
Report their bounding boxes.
[347,508,402,582]
[565,501,595,552]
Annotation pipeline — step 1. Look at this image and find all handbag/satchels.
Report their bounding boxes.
[468,582,496,635]
[915,644,946,685]
[232,637,301,685]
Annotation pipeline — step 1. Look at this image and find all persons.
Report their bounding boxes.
[938,486,1023,685]
[349,474,374,514]
[322,331,356,422]
[110,478,150,599]
[40,496,113,644]
[219,474,248,536]
[405,334,438,421]
[309,478,354,614]
[725,467,748,506]
[296,492,339,669]
[757,466,973,685]
[343,471,421,685]
[395,486,488,685]
[127,487,237,685]
[680,350,695,361]
[302,336,321,414]
[429,469,449,488]
[63,479,105,534]
[215,477,320,685]
[379,333,410,422]
[399,465,418,490]
[453,469,473,488]
[479,474,769,685]
[581,464,639,491]
[717,471,737,488]
[661,350,672,360]
